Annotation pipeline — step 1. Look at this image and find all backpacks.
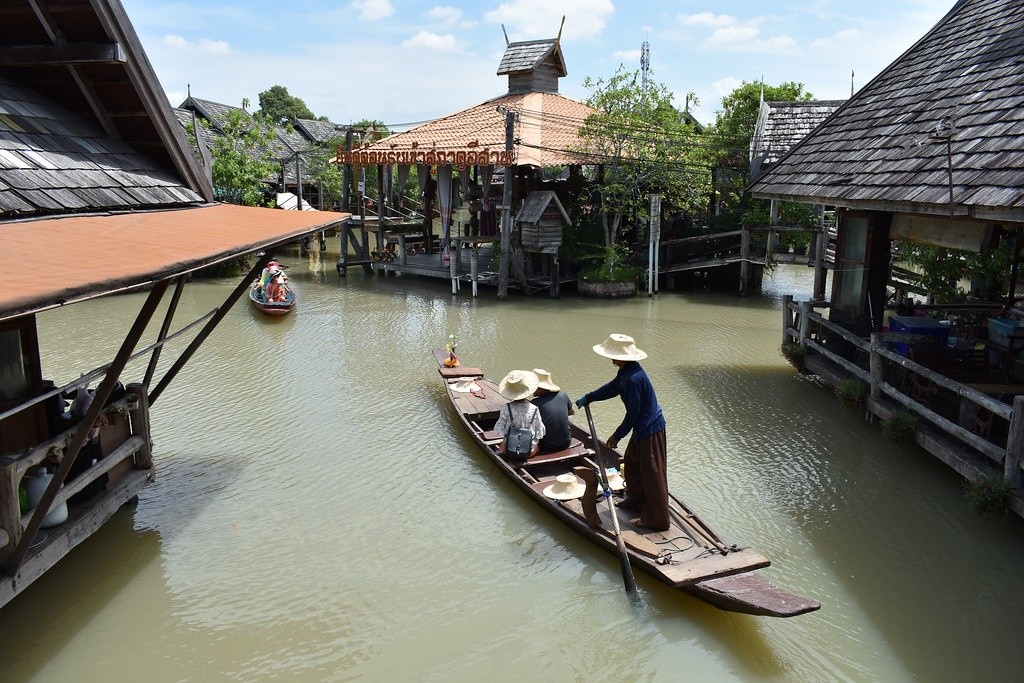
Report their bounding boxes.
[505,402,538,459]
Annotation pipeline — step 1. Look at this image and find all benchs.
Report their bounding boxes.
[484,429,596,468]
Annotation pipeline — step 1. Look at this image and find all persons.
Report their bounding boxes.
[260,258,288,303]
[494,369,574,461]
[575,334,671,532]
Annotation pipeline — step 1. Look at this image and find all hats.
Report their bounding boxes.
[269,269,281,282]
[542,474,586,500]
[449,377,482,393]
[592,333,647,361]
[597,468,627,492]
[531,368,560,392]
[498,369,539,400]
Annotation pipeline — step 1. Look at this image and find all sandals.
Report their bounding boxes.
[615,499,634,509]
[636,518,669,531]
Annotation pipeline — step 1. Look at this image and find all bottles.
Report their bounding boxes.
[27,467,67,528]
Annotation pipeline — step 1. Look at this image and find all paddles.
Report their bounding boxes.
[248,266,289,287]
[584,403,640,602]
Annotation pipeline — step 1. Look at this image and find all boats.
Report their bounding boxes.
[249,280,297,316]
[433,343,824,619]
